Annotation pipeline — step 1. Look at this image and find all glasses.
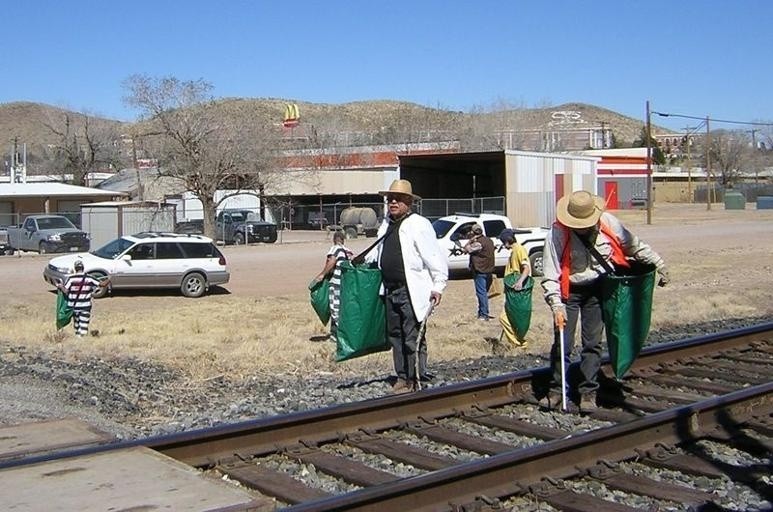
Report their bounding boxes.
[387,198,403,201]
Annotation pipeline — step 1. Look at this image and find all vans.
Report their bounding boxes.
[42,231,229,298]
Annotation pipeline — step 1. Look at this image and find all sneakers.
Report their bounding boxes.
[580,390,597,412]
[539,387,567,409]
[390,377,408,394]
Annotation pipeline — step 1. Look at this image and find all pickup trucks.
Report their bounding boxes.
[189,207,277,245]
[405,210,574,276]
[4,211,91,255]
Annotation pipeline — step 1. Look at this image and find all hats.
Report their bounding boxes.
[497,229,515,238]
[379,180,422,199]
[555,191,606,229]
[472,224,482,235]
[75,261,84,269]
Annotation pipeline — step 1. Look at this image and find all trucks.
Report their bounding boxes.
[164,189,266,245]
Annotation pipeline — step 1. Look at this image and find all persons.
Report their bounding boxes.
[316,232,355,341]
[58,260,112,337]
[364,179,449,394]
[499,228,531,350]
[540,190,670,413]
[465,224,495,321]
